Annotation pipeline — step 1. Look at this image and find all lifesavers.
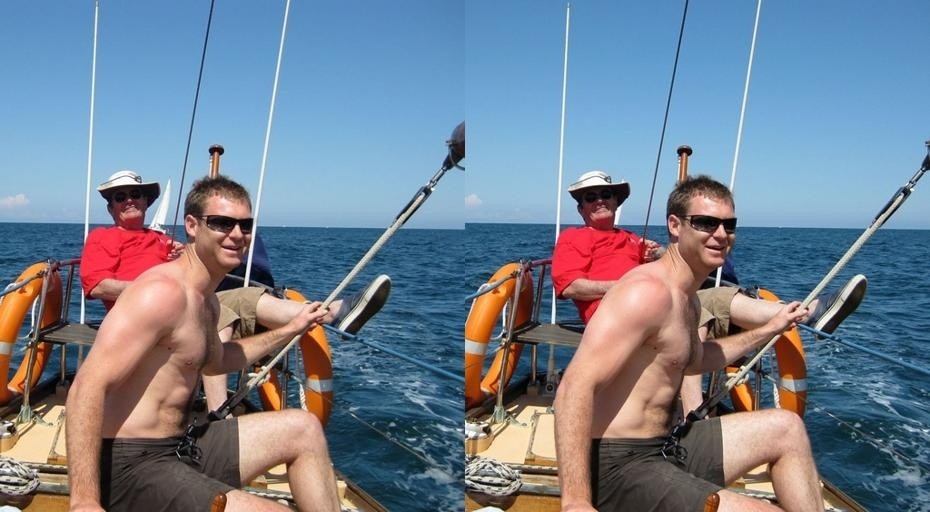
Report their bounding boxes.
[464,263,532,411]
[724,286,808,422]
[0,261,64,409]
[254,289,331,430]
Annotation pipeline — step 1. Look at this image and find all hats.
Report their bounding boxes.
[567,171,630,208]
[98,169,161,207]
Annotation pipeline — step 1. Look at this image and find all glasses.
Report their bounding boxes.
[679,215,737,233]
[113,188,142,202]
[201,214,254,235]
[586,189,614,204]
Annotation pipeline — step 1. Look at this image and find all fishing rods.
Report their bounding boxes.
[547,2,764,388]
[75,1,291,376]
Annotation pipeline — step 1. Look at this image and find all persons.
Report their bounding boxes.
[64,175,342,512]
[552,175,826,512]
[550,170,868,423]
[78,170,392,422]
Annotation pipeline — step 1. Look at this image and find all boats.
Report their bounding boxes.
[465,257,930,512]
[1,256,464,512]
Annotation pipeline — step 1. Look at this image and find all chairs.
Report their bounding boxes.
[18,257,105,421]
[490,258,587,423]
